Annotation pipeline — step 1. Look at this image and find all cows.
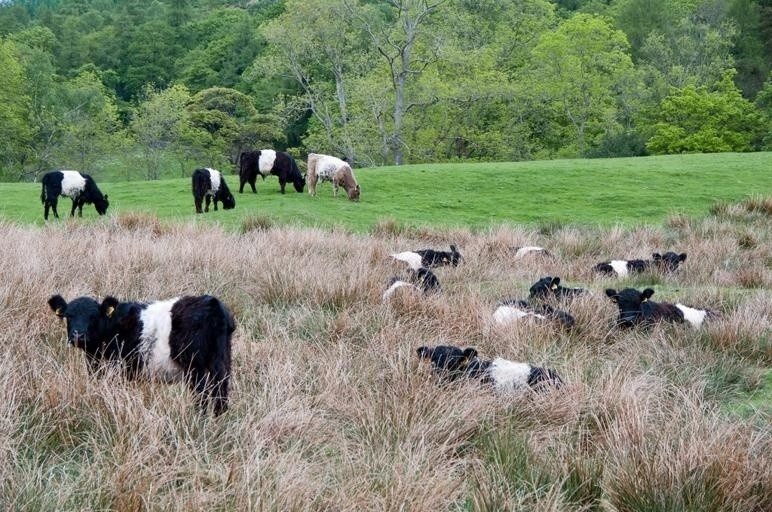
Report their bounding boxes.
[47,294,236,417]
[592,252,687,280]
[384,245,466,270]
[415,344,563,396]
[527,276,593,299]
[192,167,235,213]
[604,287,721,332]
[382,270,441,304]
[239,149,307,194]
[307,153,360,202]
[493,299,574,335]
[505,245,555,264]
[41,170,109,219]
